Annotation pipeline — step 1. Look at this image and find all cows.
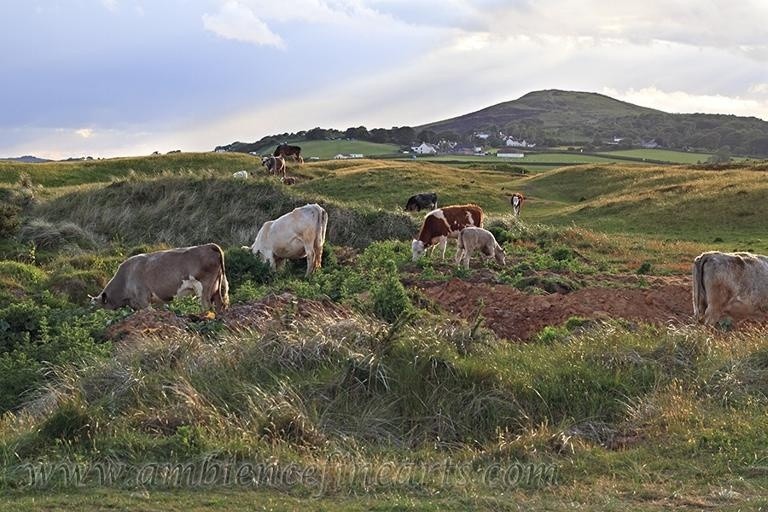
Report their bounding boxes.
[232,170,247,178]
[412,203,484,263]
[404,192,437,212]
[261,157,286,177]
[454,227,506,270]
[273,143,303,164]
[280,177,296,186]
[240,203,328,279]
[510,192,523,216]
[87,243,229,311]
[691,250,768,327]
